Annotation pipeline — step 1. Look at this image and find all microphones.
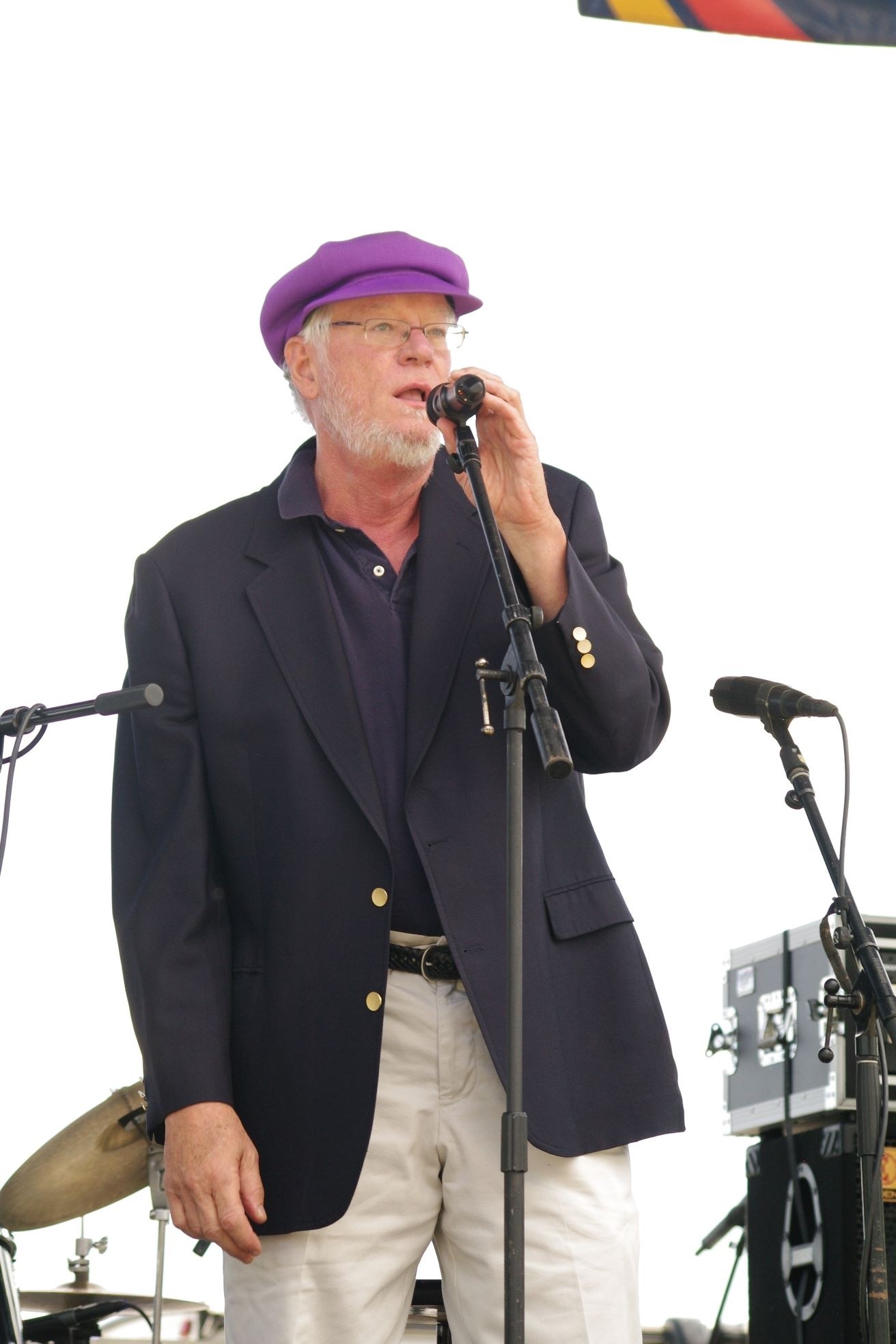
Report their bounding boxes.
[424,374,488,426]
[21,1302,134,1337]
[709,677,838,720]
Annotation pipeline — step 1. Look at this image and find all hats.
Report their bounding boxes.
[260,231,484,368]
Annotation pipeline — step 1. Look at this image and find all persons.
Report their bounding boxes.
[108,234,688,1344]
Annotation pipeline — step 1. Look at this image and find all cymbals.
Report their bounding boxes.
[0,1080,151,1231]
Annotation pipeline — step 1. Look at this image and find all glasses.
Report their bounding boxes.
[324,315,470,353]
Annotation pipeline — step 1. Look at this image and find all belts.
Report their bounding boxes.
[386,941,467,984]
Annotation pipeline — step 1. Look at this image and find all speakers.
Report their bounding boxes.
[748,1110,896,1343]
